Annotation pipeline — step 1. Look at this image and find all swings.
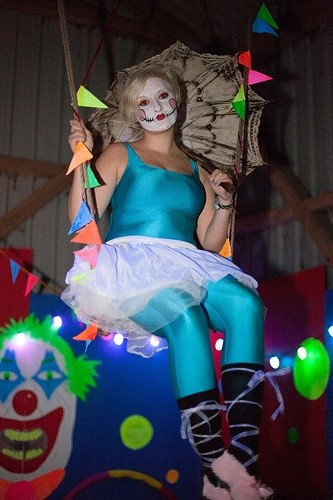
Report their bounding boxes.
[56,0,268,336]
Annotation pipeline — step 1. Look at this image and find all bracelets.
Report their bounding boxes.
[210,198,235,211]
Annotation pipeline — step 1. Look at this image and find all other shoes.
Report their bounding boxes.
[202,475,274,500]
[212,449,265,500]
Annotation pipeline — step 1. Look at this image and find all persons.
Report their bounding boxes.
[59,66,273,500]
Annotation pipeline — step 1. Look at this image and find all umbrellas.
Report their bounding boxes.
[88,40,269,194]
